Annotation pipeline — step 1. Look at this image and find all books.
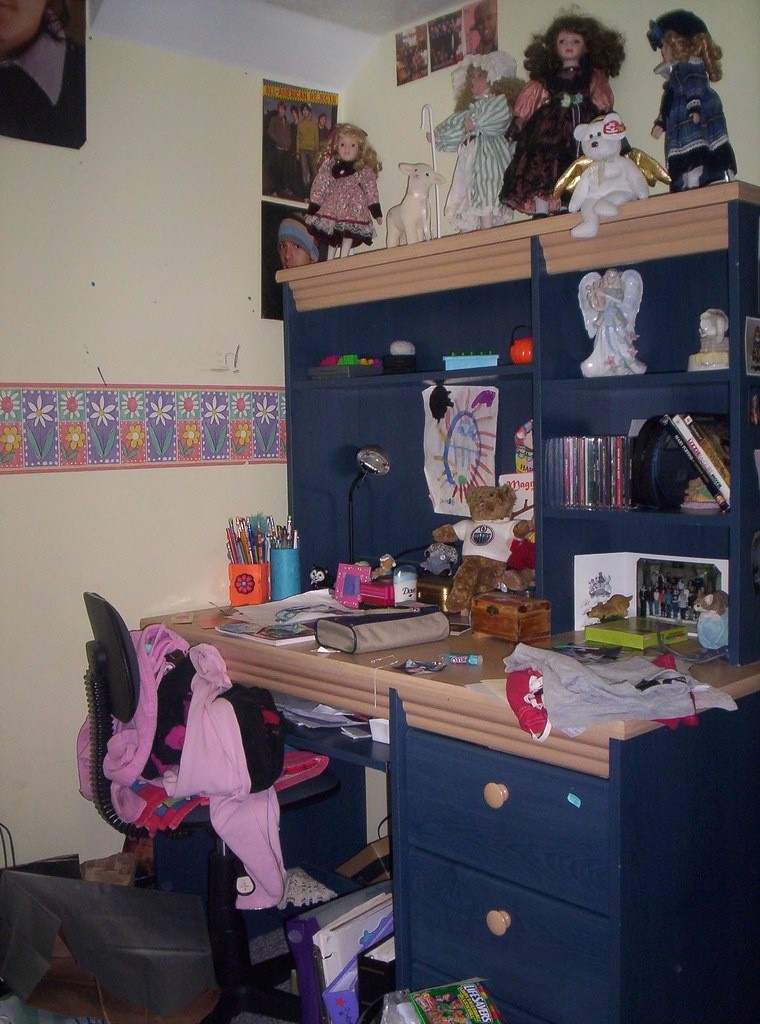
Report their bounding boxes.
[215,621,317,647]
[660,411,730,514]
[543,434,633,510]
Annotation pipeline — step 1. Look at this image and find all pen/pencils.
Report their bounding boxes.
[224,512,301,564]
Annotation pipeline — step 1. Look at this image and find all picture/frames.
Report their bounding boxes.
[333,563,371,609]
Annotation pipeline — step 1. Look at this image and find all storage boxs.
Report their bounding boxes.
[585,617,688,650]
[335,836,391,886]
[470,591,553,645]
[361,582,394,606]
[418,578,455,614]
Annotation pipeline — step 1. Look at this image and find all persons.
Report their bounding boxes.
[500,15,627,219]
[278,211,322,270]
[582,268,647,375]
[751,394,760,426]
[268,101,330,202]
[427,51,521,230]
[646,9,738,191]
[397,0,497,84]
[0,0,86,148]
[304,124,383,258]
[638,572,703,619]
[752,327,760,371]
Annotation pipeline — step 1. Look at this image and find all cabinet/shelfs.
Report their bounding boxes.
[274,182,760,663]
[139,606,760,1024]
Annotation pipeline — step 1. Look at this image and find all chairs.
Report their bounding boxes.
[81,589,341,1024]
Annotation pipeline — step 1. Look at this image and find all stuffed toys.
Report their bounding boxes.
[432,484,529,612]
[587,595,633,623]
[693,589,729,621]
[553,113,672,236]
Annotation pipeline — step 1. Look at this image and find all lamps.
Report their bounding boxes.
[348,445,390,564]
